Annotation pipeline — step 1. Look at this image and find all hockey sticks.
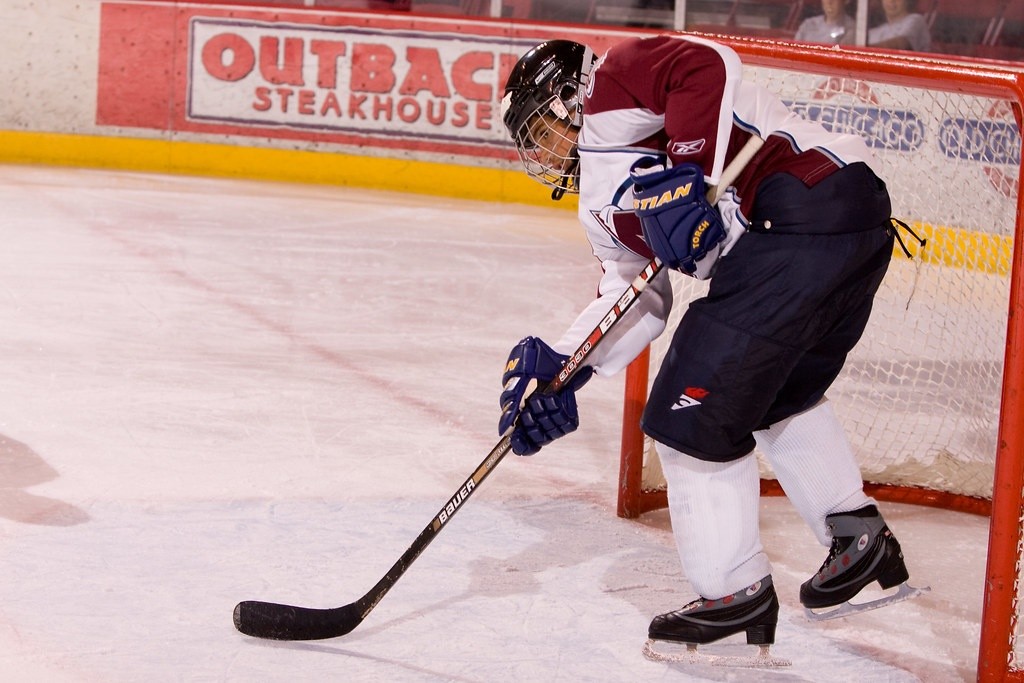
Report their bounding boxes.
[234,135,766,641]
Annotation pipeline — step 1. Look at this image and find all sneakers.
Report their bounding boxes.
[645,573,791,673]
[800,506,933,622]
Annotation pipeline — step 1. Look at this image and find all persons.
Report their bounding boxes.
[498,33,934,670]
[794,1,931,51]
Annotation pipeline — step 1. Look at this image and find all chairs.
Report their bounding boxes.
[675,0,1024,64]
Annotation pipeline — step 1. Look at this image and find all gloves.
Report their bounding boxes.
[628,156,726,273]
[497,335,594,456]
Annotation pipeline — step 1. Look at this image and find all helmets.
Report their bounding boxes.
[503,39,599,148]
